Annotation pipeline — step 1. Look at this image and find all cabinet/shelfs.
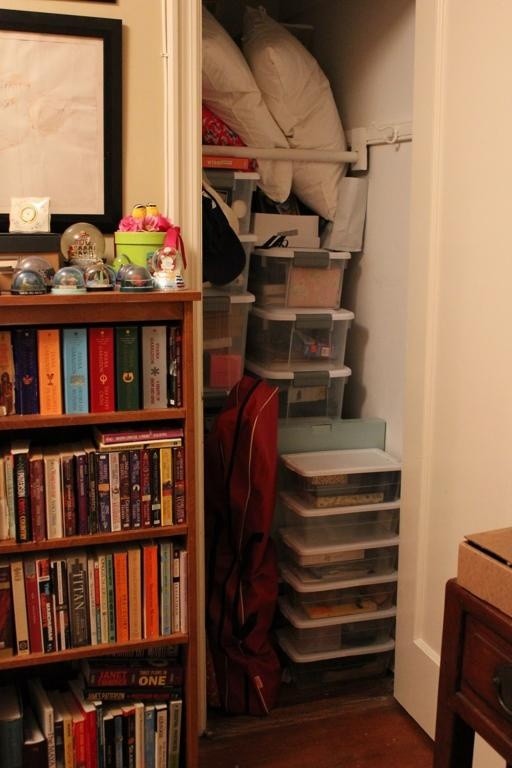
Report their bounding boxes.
[0,291,201,768]
[433,575,512,767]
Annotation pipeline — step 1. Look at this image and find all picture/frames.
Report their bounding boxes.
[0,0,123,233]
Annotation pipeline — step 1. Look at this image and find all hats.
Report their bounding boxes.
[202,181,246,284]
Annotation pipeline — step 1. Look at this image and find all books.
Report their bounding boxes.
[1,542,187,656]
[1,325,181,416]
[1,422,185,542]
[284,473,398,686]
[1,658,182,768]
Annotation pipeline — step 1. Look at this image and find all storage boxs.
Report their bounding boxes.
[202,169,400,704]
[456,527,512,619]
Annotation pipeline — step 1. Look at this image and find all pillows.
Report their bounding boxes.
[202,5,349,223]
